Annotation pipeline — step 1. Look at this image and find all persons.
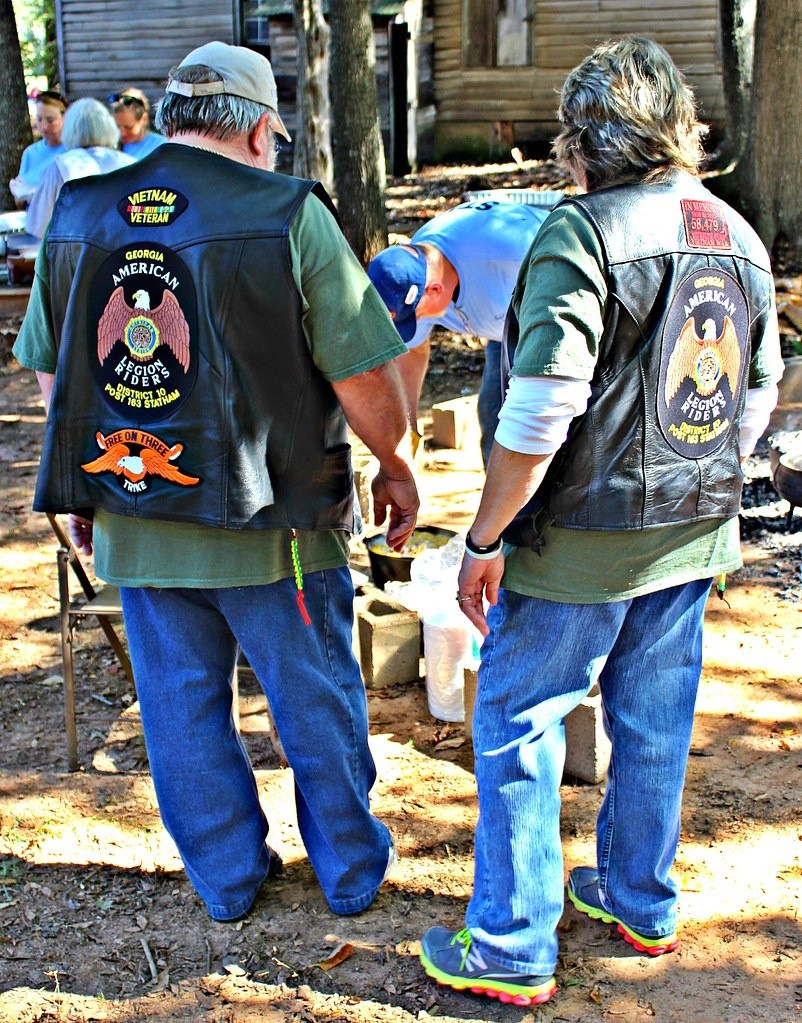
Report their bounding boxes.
[418,38,785,1009]
[8,88,167,243]
[12,42,419,922]
[368,192,562,471]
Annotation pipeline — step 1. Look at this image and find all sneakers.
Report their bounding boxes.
[419,925,557,1006]
[568,866,679,956]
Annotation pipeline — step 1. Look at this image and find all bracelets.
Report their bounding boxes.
[465,530,504,561]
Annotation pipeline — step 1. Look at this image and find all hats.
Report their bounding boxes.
[165,41,292,142]
[367,244,427,343]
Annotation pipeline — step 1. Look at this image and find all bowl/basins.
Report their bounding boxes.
[361,524,459,592]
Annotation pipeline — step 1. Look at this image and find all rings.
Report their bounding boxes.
[456,596,471,601]
[81,524,92,529]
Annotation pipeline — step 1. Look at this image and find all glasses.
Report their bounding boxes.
[108,93,144,107]
[36,91,69,107]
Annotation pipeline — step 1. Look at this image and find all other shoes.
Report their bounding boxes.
[210,831,283,922]
[383,846,398,882]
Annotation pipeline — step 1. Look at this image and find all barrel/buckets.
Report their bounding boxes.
[417,610,489,722]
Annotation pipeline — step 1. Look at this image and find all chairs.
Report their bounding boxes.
[44,511,253,771]
